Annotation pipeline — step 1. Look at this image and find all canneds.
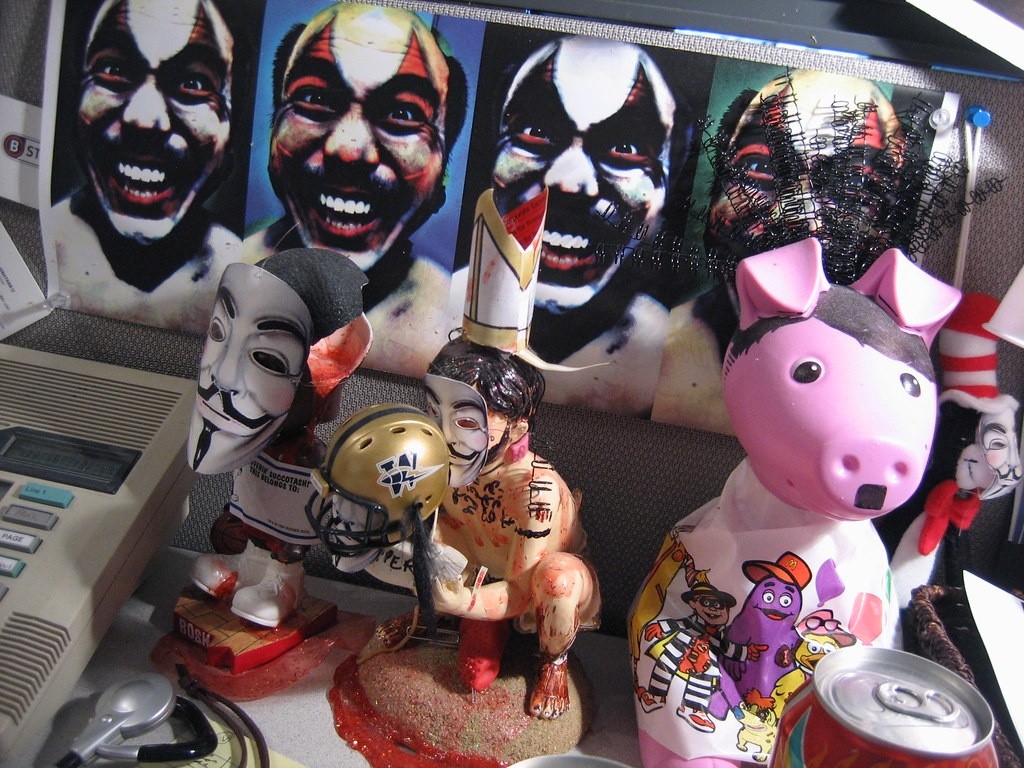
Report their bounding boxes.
[767,646,1000,768]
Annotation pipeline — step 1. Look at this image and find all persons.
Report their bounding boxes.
[54,0,910,435]
[185,245,370,629]
[330,338,601,718]
